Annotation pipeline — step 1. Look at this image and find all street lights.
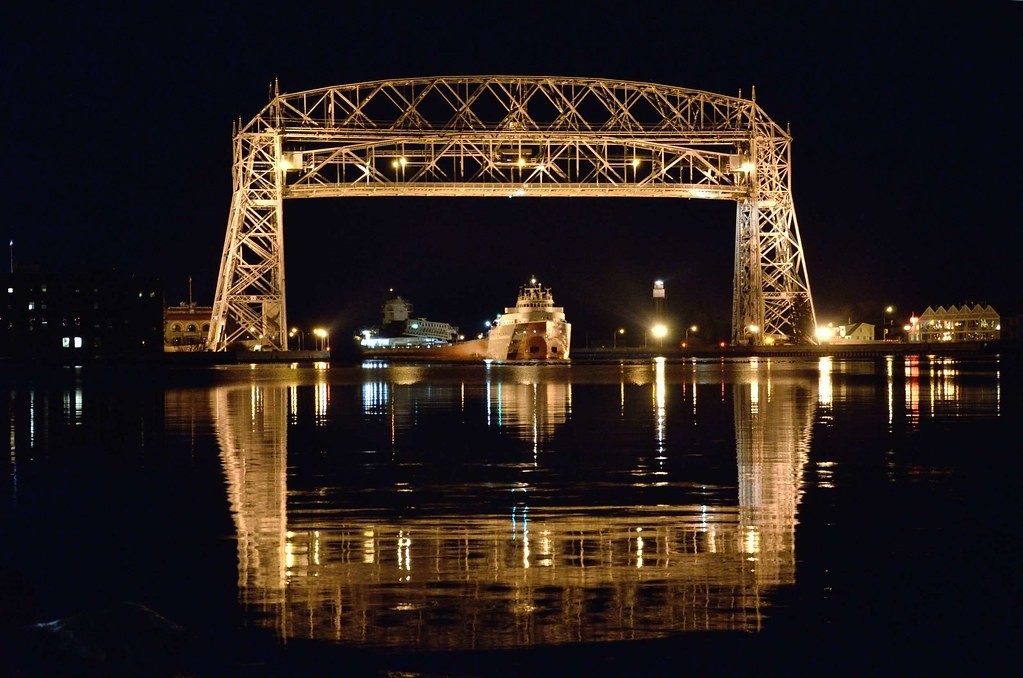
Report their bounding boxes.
[883,306,893,340]
[288,324,329,353]
[686,326,696,346]
[645,328,656,348]
[613,329,625,350]
